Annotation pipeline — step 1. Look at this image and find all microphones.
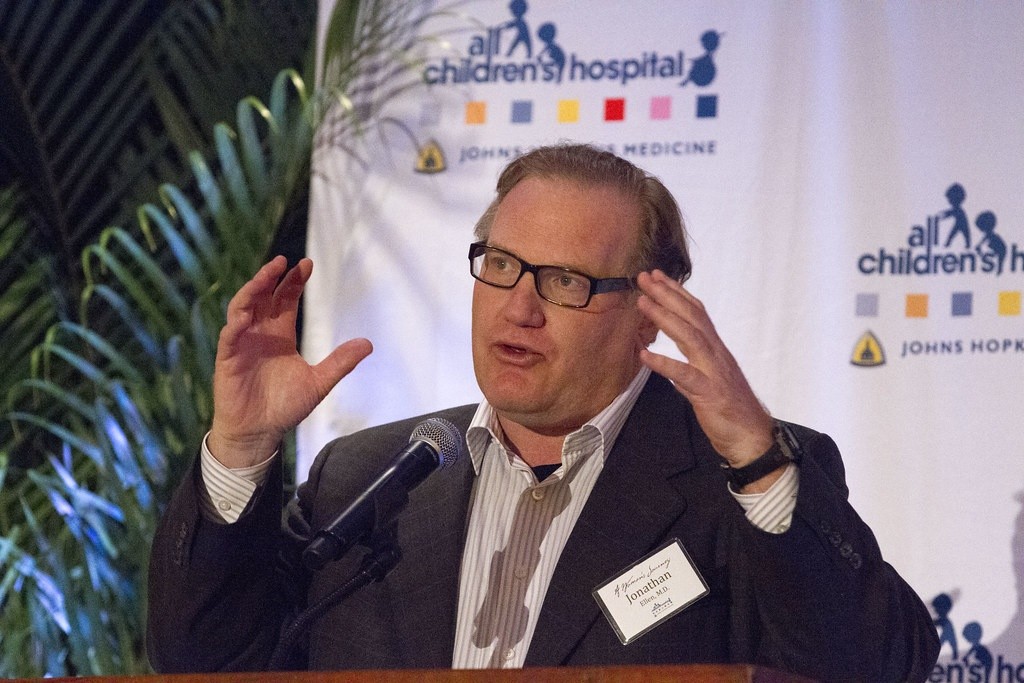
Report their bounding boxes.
[301,417,462,571]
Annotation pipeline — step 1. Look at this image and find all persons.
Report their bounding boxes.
[143,143,941,683]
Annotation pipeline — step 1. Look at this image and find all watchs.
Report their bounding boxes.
[720,418,802,490]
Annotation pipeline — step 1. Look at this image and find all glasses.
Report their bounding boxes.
[468,240,639,307]
